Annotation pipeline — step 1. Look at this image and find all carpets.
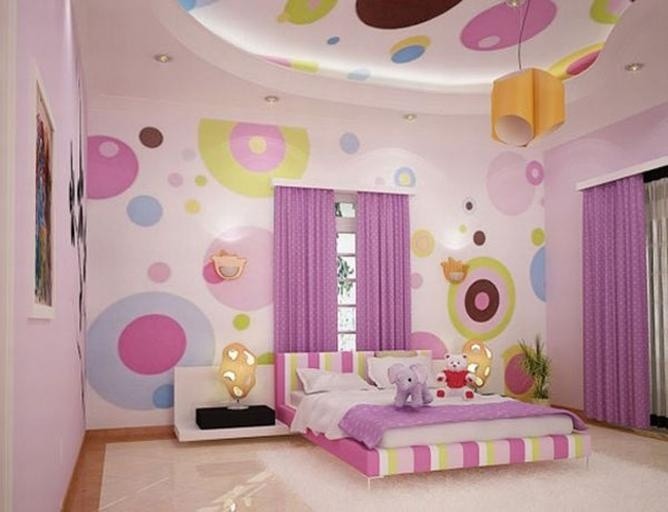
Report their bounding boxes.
[97,423,668,512]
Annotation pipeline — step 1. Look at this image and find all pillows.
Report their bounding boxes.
[296,354,432,394]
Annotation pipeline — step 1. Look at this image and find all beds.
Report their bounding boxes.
[277,346,592,487]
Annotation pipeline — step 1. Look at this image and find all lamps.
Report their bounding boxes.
[490,1,566,145]
[219,341,258,410]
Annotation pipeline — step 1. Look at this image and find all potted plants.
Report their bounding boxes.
[516,331,553,406]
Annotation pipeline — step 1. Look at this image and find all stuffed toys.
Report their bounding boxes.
[387,363,434,409]
[434,352,477,401]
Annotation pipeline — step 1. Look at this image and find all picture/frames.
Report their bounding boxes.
[31,62,54,321]
